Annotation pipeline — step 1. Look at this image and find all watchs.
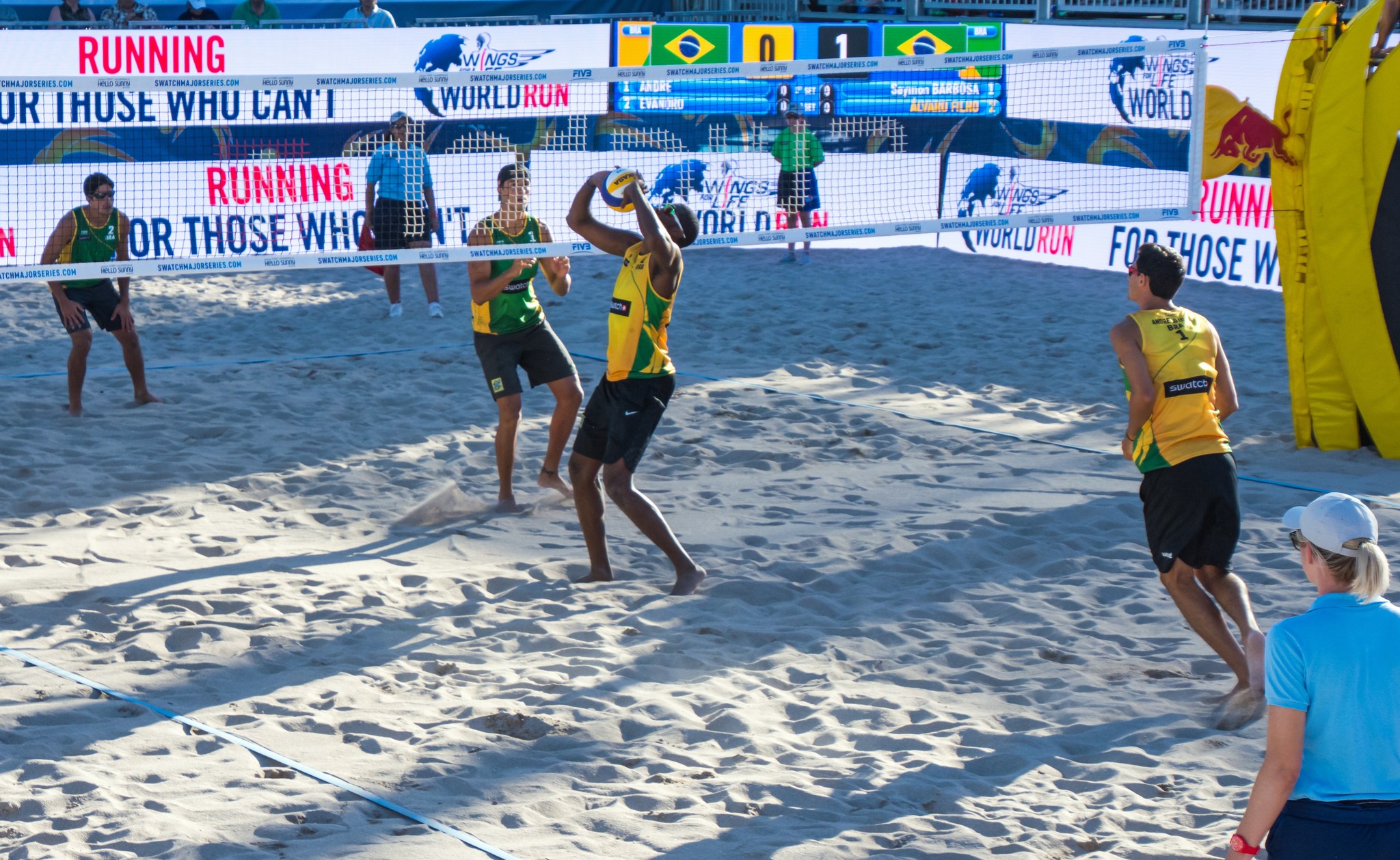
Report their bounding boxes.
[1229,834,1261,855]
[429,212,439,218]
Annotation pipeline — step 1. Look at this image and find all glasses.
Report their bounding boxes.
[1289,530,1316,554]
[786,114,802,119]
[1129,264,1142,276]
[393,123,410,130]
[87,190,115,199]
[661,204,686,238]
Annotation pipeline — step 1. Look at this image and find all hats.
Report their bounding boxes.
[389,110,413,124]
[1282,491,1378,558]
[785,102,803,115]
[188,0,206,9]
[498,163,531,201]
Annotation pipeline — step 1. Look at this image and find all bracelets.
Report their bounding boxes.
[585,174,597,189]
[1126,429,1135,442]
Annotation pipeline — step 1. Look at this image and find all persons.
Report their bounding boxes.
[1222,487,1400,860]
[364,111,450,319]
[231,0,282,30]
[101,0,162,29]
[49,0,98,30]
[1108,242,1271,702]
[771,100,825,268]
[339,0,400,29]
[174,0,223,29]
[40,172,168,412]
[568,165,708,595]
[466,163,586,513]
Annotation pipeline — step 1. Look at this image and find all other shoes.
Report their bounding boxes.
[776,253,796,265]
[798,251,812,266]
[389,304,402,316]
[427,303,444,318]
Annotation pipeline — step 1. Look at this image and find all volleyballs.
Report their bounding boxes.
[601,167,639,213]
